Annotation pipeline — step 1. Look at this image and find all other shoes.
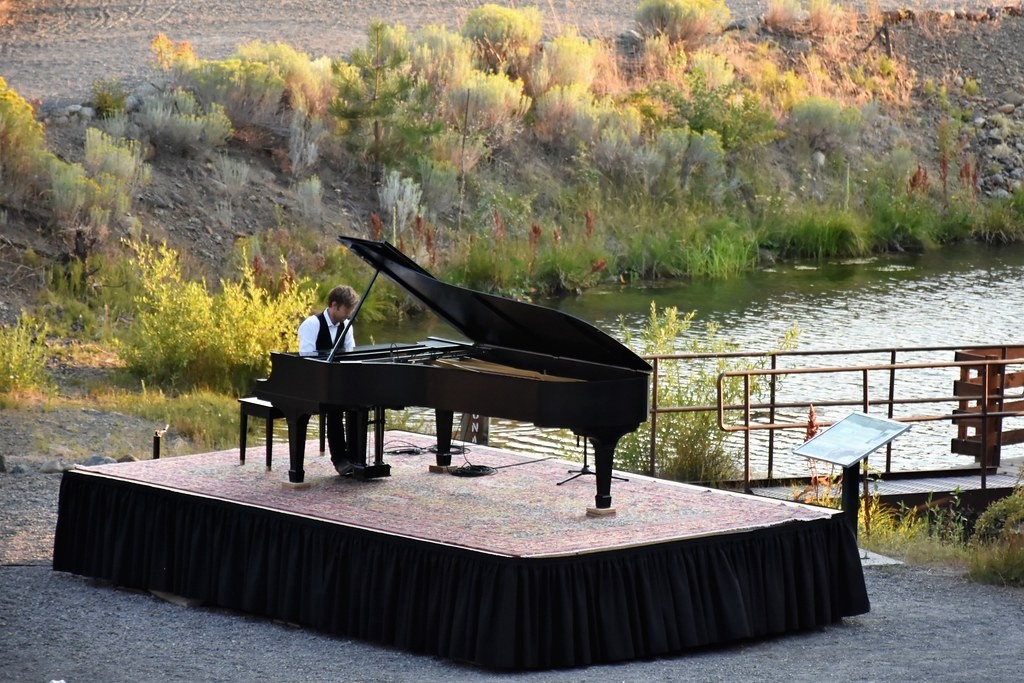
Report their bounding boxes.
[336,458,353,475]
[354,461,367,470]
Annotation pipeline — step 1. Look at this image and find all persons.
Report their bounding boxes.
[297,286,369,476]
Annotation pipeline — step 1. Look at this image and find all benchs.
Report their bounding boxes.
[237,397,325,472]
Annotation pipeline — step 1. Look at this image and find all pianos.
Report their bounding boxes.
[253,234,652,509]
